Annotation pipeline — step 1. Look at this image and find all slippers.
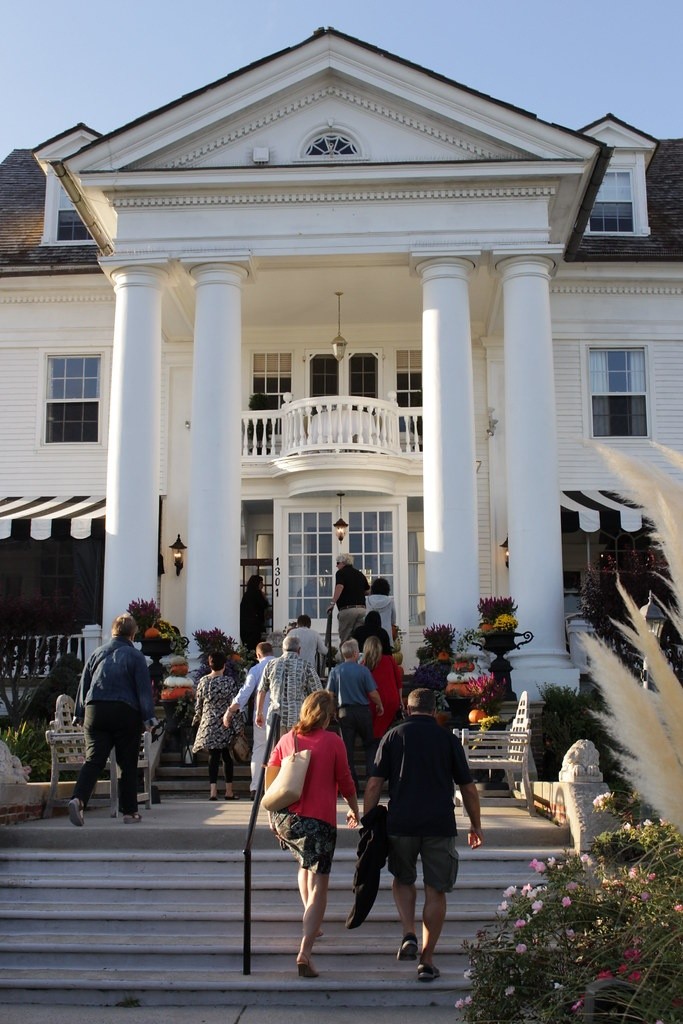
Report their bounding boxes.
[209,796,217,801]
[224,795,240,800]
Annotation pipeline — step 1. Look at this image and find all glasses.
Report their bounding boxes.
[337,562,343,565]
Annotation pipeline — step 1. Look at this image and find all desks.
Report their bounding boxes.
[311,411,377,452]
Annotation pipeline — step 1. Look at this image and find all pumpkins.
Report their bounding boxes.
[438,651,448,660]
[231,652,240,660]
[144,623,159,638]
[161,656,194,700]
[468,709,486,723]
[445,661,479,696]
[481,624,494,631]
[435,712,448,725]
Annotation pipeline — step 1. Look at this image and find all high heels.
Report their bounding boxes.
[297,951,319,977]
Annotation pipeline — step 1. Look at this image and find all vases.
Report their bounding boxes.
[138,637,191,661]
[445,695,473,730]
[159,699,188,753]
[465,631,534,658]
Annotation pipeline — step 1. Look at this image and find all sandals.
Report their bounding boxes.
[68,797,84,826]
[123,814,142,824]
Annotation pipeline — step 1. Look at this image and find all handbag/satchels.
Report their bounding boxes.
[261,728,312,812]
[229,732,252,765]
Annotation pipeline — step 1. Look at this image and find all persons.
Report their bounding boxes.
[326,553,371,662]
[69,613,154,825]
[240,574,272,651]
[297,576,325,617]
[264,689,360,977]
[191,651,246,801]
[363,687,485,981]
[224,643,276,800]
[256,578,404,799]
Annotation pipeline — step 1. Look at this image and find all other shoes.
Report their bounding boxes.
[417,963,440,979]
[396,934,418,961]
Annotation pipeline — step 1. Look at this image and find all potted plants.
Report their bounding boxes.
[245,393,271,455]
[409,416,423,453]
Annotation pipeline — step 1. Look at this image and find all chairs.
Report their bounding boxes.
[453,690,536,818]
[304,415,382,443]
[44,694,152,819]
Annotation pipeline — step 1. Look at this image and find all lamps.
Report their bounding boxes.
[500,534,509,570]
[331,291,347,362]
[484,409,498,439]
[169,534,187,577]
[333,492,349,543]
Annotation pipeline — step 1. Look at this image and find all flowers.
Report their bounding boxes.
[129,598,172,640]
[191,627,257,689]
[390,595,519,750]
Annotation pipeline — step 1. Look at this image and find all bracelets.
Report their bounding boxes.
[330,602,335,604]
[257,712,263,716]
[227,707,235,713]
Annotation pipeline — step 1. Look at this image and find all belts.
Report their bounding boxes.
[340,605,364,610]
